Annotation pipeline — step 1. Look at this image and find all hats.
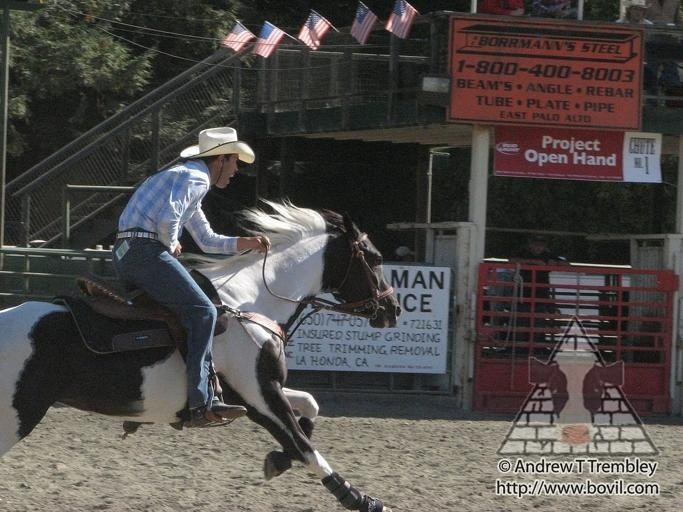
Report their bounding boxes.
[179,126,256,165]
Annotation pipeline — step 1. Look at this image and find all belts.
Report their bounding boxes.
[115,231,158,241]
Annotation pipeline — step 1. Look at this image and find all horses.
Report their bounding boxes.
[0,193,403,512]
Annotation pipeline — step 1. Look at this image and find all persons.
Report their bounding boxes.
[509,232,569,360]
[109,127,270,430]
[477,0,524,17]
[616,0,653,25]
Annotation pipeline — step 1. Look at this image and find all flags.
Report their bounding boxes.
[251,20,284,58]
[349,2,378,46]
[385,0,418,39]
[297,9,331,50]
[223,22,255,53]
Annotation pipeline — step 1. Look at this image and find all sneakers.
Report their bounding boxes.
[192,401,248,425]
